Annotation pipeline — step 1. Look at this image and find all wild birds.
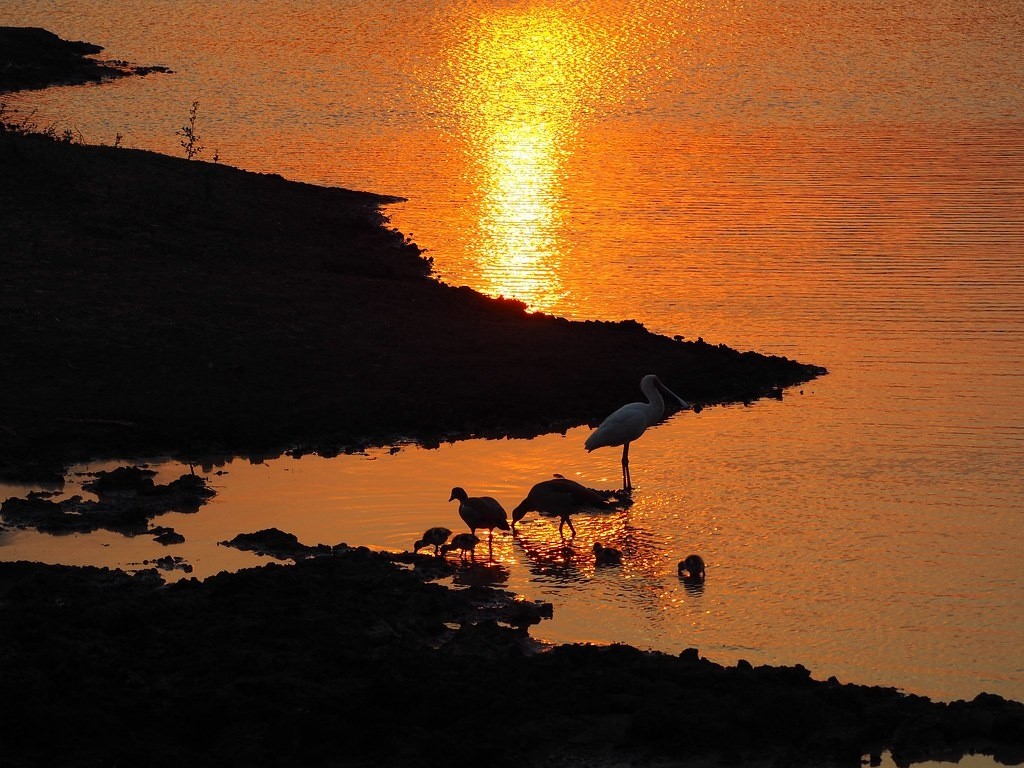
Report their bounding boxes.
[449,487,510,534]
[440,533,480,557]
[591,542,623,563]
[413,527,453,553]
[585,374,689,477]
[677,555,705,578]
[511,479,617,534]
